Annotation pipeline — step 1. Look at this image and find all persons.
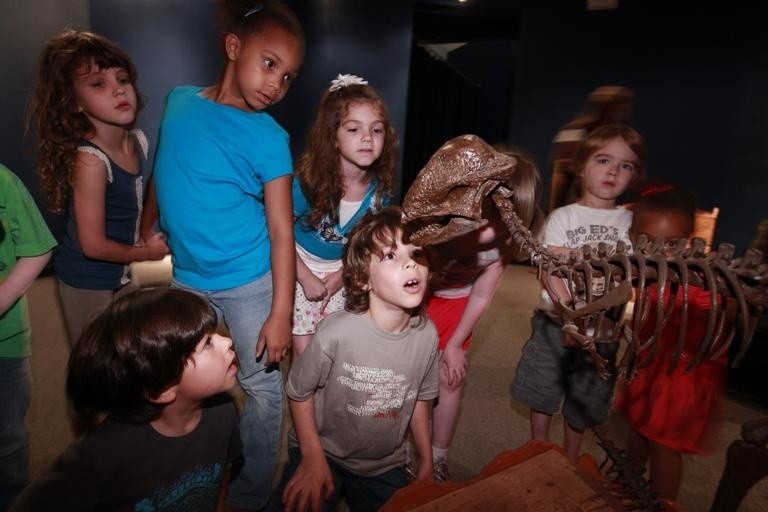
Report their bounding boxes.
[18,30,171,351]
[604,183,744,512]
[272,204,441,511]
[136,1,308,510]
[544,83,637,216]
[2,285,244,511]
[0,164,60,512]
[507,122,651,470]
[402,147,544,489]
[286,72,401,460]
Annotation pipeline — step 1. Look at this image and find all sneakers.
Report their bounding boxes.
[434,455,451,483]
[404,452,418,480]
